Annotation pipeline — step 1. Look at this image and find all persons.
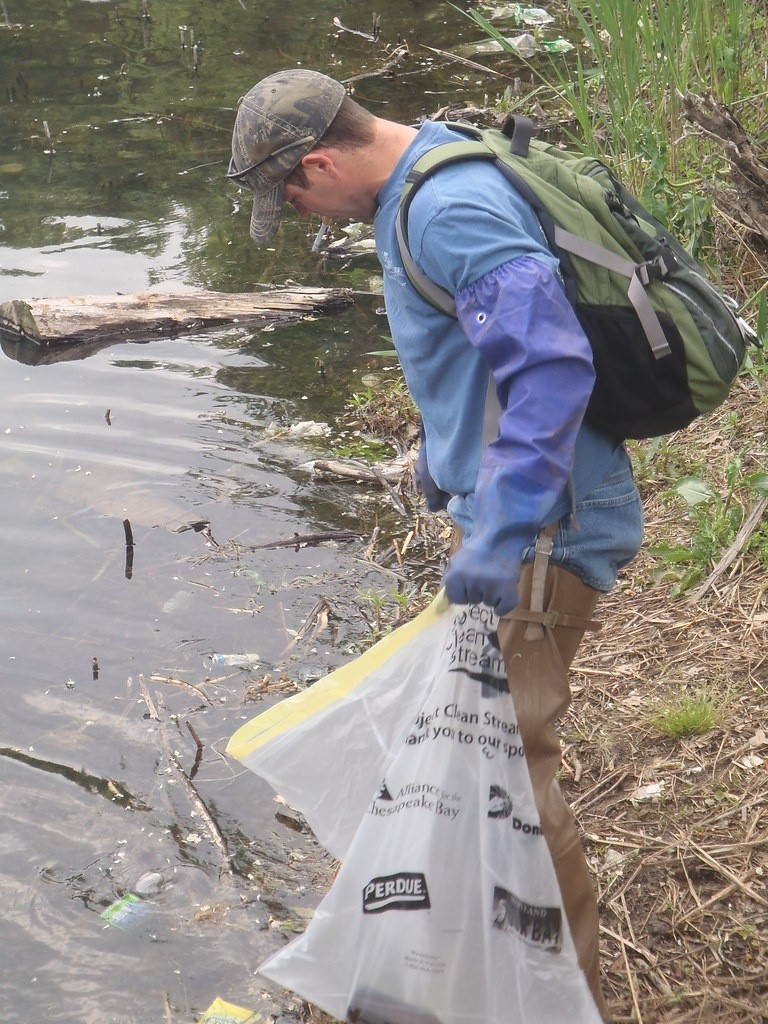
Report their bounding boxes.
[225,69,645,1023]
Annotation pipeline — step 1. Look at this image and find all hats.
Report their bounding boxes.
[231,69,347,244]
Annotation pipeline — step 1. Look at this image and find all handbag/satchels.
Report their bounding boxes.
[226,585,606,1024]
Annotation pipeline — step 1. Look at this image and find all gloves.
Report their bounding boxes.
[414,420,452,512]
[444,251,597,616]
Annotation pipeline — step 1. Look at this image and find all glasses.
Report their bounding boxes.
[225,136,314,191]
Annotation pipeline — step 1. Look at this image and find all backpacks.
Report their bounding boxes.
[396,115,763,440]
